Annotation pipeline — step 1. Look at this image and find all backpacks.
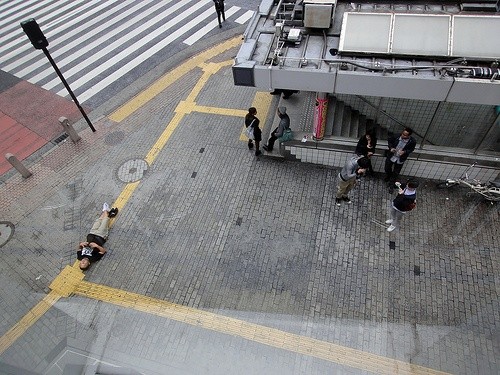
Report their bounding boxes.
[278,122,294,143]
[406,199,416,211]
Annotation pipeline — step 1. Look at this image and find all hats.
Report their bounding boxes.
[279,105,287,114]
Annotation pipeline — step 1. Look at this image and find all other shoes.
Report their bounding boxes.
[270,91,281,96]
[108,208,118,218]
[335,198,342,206]
[283,93,293,99]
[341,196,352,204]
[248,144,254,149]
[255,150,262,157]
[263,144,273,152]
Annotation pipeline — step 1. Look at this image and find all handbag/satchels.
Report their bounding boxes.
[242,119,257,140]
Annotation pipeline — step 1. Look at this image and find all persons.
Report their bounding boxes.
[262,106,289,151]
[76,203,112,270]
[335,155,370,206]
[213,0,225,28]
[384,127,417,189]
[244,107,262,157]
[386,178,419,232]
[356,132,377,180]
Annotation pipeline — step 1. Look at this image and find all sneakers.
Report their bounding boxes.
[385,218,394,223]
[387,225,397,232]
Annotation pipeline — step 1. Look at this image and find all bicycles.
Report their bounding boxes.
[436,161,500,206]
